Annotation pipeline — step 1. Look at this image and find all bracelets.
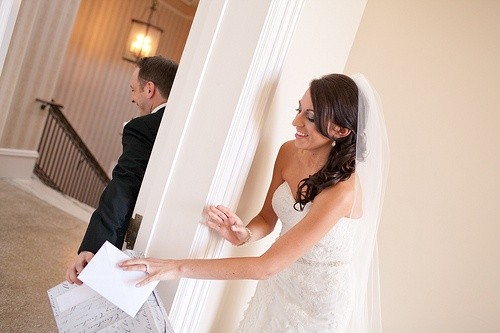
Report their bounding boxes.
[233,227,252,247]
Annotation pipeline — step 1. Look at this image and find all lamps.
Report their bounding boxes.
[122,0,163,64]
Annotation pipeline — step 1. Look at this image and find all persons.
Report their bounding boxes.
[119,73,390,333]
[67,55,177,285]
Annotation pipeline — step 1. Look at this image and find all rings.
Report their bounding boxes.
[145,265,150,273]
